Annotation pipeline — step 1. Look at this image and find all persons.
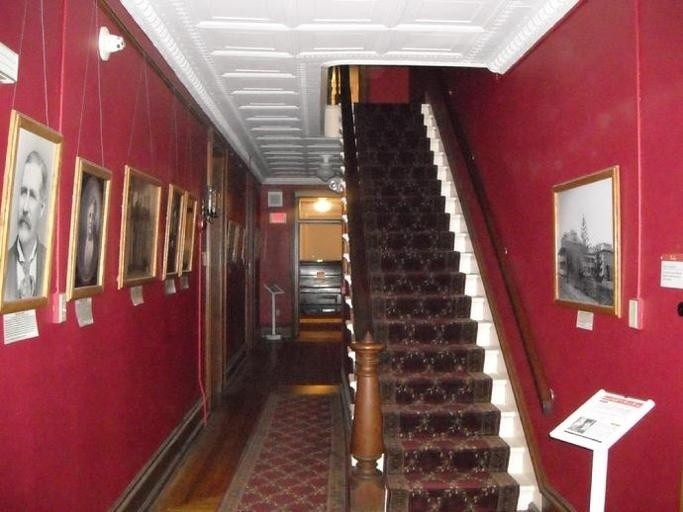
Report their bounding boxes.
[5,152,46,300]
[78,195,98,281]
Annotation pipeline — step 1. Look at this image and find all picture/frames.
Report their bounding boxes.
[551,165,622,318]
[0,110,65,314]
[65,157,114,303]
[117,164,247,291]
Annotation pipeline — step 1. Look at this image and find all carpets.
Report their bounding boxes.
[218,384,349,512]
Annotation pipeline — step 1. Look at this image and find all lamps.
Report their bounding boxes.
[317,155,335,182]
